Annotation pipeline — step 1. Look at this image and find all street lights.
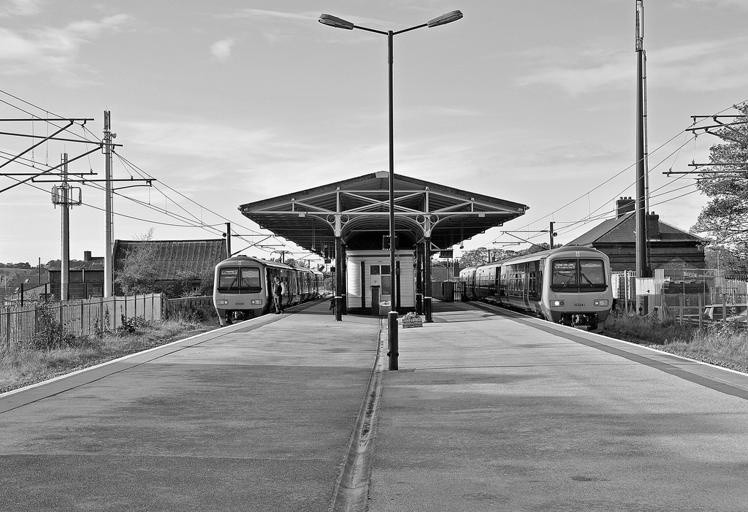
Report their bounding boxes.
[316,9,463,368]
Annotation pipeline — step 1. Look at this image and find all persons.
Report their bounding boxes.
[273,276,285,314]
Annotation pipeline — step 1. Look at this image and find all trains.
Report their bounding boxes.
[458,244,613,334]
[213,254,325,326]
